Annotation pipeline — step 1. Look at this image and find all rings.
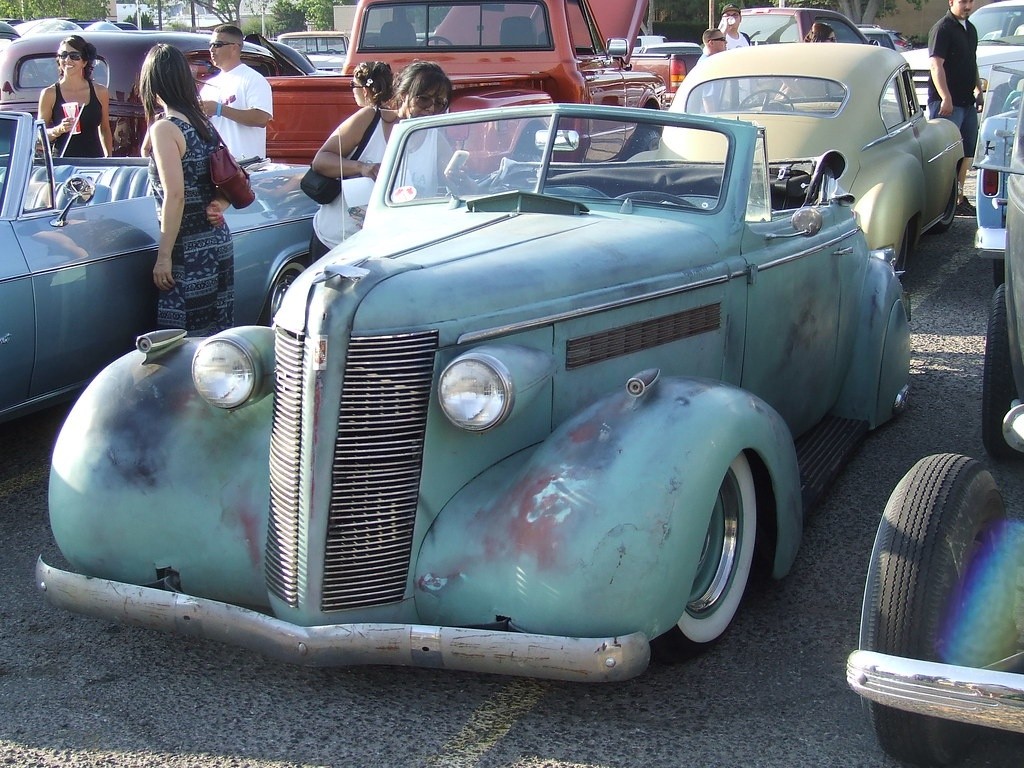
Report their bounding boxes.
[61,127,65,132]
[163,280,166,283]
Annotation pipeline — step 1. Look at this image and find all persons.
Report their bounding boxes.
[720,3,757,109]
[36,35,112,158]
[350,62,394,108]
[780,22,837,98]
[928,0,985,217]
[696,28,728,114]
[196,25,274,162]
[308,61,479,273]
[139,44,235,336]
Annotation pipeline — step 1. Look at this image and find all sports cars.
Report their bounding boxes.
[2,107,335,428]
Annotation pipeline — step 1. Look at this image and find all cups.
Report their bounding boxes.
[728,15,736,26]
[62,102,82,135]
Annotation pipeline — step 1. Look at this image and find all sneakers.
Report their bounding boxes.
[954,196,976,217]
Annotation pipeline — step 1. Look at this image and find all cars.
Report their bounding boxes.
[0,18,320,165]
[893,1,1024,142]
[25,101,920,684]
[632,33,703,58]
[267,0,666,184]
[859,23,915,53]
[717,10,881,49]
[278,26,434,79]
[840,146,1024,765]
[619,39,968,277]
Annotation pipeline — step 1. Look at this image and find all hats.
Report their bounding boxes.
[721,3,741,16]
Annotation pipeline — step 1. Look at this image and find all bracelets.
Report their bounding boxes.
[217,104,221,117]
[52,127,60,138]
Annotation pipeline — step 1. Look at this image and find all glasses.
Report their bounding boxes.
[349,80,372,91]
[409,91,449,115]
[824,36,837,42]
[722,13,740,18]
[708,36,726,43]
[57,51,82,61]
[209,41,241,48]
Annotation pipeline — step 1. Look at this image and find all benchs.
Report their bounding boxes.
[523,167,812,211]
[748,104,904,129]
[19,166,156,216]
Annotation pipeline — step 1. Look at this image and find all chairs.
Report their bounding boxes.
[1000,15,1024,37]
[499,16,538,47]
[56,181,112,210]
[1000,89,1022,114]
[380,20,416,47]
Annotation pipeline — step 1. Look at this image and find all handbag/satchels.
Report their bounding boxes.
[301,107,382,204]
[202,115,255,210]
